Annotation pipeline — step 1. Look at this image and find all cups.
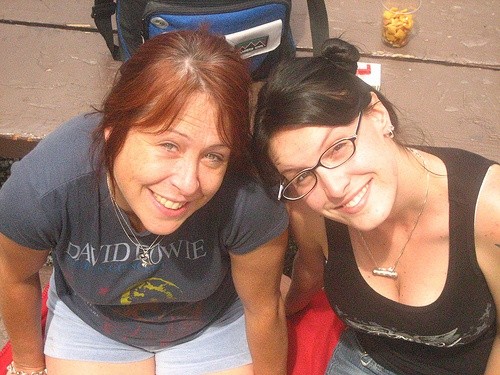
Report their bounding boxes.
[380,0,422,48]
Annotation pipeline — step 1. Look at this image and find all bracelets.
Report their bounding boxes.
[6,361,47,375]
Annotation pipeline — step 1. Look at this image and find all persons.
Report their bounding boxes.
[1,28,289,374]
[253,34,498,375]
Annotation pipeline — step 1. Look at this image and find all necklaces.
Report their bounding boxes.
[99,169,165,268]
[357,149,430,279]
